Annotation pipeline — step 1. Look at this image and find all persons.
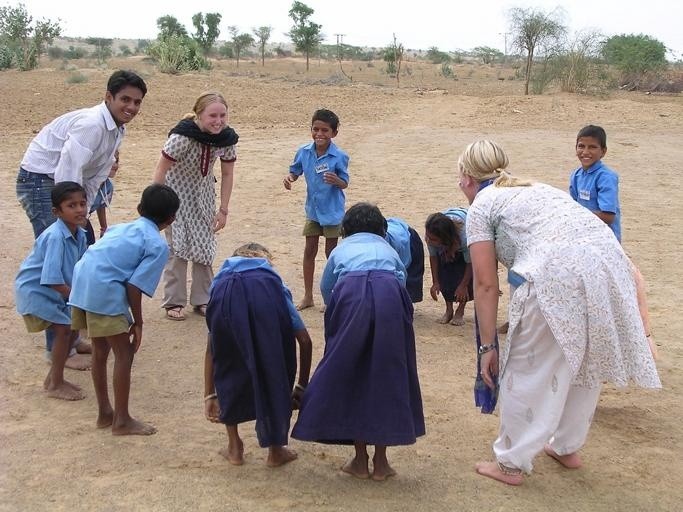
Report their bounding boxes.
[66,184,180,436]
[87,148,120,237]
[568,125,620,247]
[425,206,473,327]
[380,218,424,304]
[287,201,426,481]
[283,108,348,313]
[205,243,313,468]
[154,89,239,319]
[496,267,527,335]
[12,180,89,403]
[457,139,663,486]
[15,68,148,373]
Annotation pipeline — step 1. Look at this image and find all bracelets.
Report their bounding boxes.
[478,343,498,356]
[204,392,218,401]
[217,206,228,217]
[295,382,309,394]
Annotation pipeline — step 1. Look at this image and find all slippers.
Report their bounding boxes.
[166,304,209,321]
